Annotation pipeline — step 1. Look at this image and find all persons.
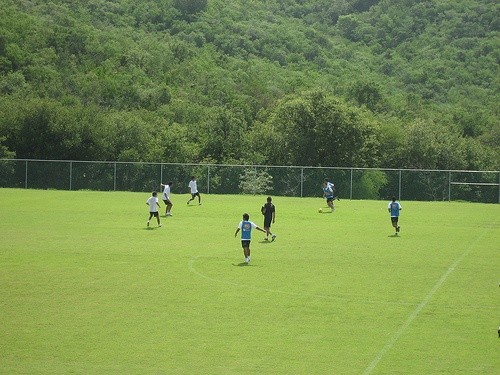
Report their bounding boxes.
[235,213,266,264]
[146,191,162,227]
[261,197,277,242]
[324,178,335,201]
[323,181,335,212]
[161,182,173,216]
[387,196,402,236]
[187,176,203,205]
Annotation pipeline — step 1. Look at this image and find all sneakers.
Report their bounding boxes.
[158,225,162,227]
[245,256,250,263]
[147,221,149,226]
[271,235,276,241]
[264,238,268,240]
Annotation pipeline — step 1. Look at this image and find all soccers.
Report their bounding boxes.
[318,208,322,213]
